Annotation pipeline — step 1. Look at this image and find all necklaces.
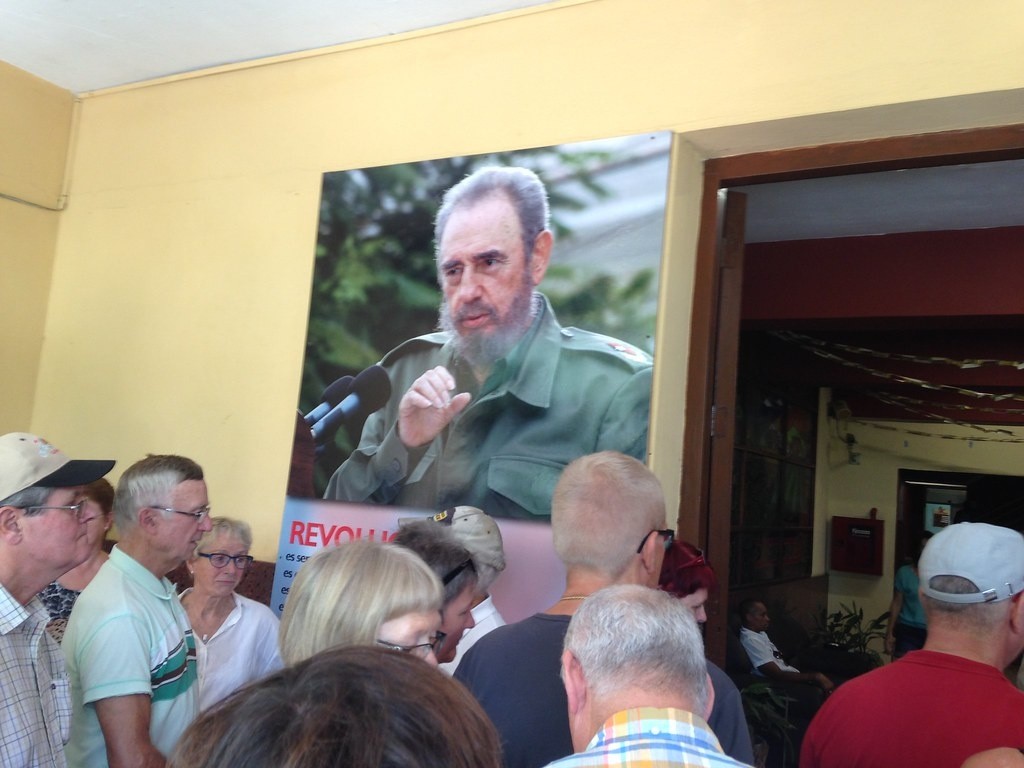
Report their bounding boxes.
[562,596,586,600]
[187,595,236,641]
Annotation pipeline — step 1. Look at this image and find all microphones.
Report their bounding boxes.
[304,366,391,446]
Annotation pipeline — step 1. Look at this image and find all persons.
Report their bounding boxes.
[35,478,115,645]
[177,516,285,711]
[60,454,212,768]
[278,540,449,668]
[0,432,116,768]
[541,584,756,768]
[739,599,833,719]
[398,506,507,676]
[391,519,479,664]
[322,166,653,523]
[166,645,502,768]
[883,531,934,662]
[451,451,754,768]
[657,539,715,623]
[799,522,1024,768]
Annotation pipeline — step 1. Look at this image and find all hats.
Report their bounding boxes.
[919,521,1024,604]
[0,432,116,503]
[398,504,504,571]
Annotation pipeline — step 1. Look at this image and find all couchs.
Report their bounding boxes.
[727,614,870,736]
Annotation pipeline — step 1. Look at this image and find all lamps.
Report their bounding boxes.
[827,399,851,420]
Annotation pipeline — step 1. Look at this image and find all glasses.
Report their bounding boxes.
[680,549,706,569]
[637,528,674,555]
[23,497,88,518]
[151,506,210,523]
[442,549,475,586]
[374,630,447,660]
[198,552,253,569]
